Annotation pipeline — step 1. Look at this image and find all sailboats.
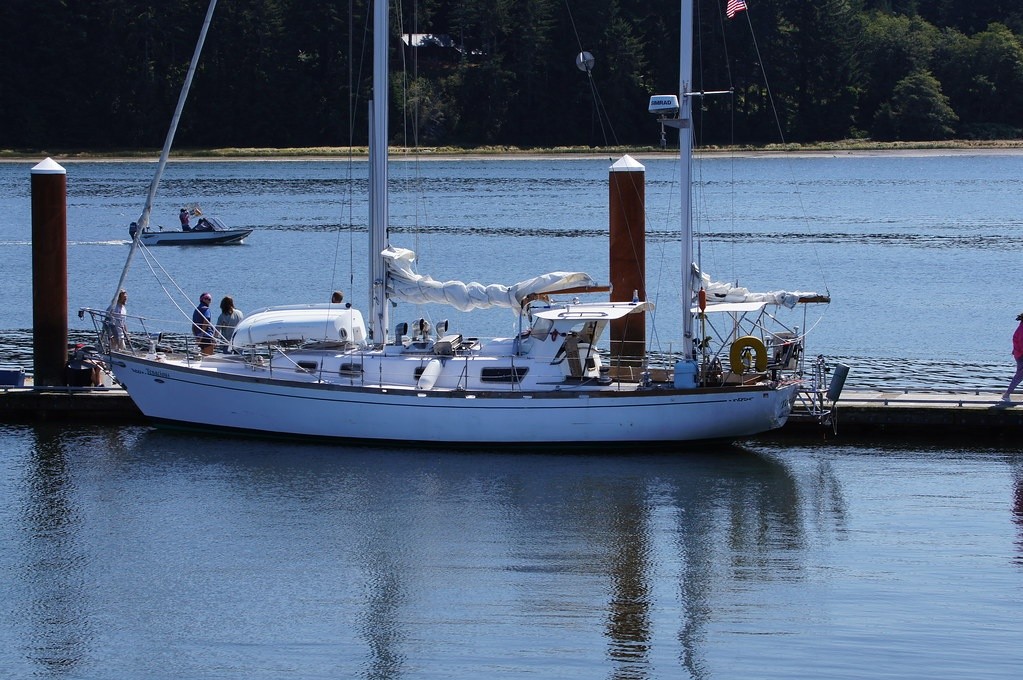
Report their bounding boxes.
[89,0,856,454]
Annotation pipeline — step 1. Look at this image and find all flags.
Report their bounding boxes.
[726,0,745,19]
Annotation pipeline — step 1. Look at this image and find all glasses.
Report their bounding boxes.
[205,299,211,302]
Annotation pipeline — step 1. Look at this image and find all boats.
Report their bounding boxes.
[127,216,254,246]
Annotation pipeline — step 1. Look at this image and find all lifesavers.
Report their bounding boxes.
[729,335,768,375]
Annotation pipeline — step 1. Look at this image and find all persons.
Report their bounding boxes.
[332,291,343,303]
[1002,313,1023,400]
[180,208,191,231]
[106,290,129,351]
[198,219,206,230]
[213,297,243,354]
[192,293,215,354]
